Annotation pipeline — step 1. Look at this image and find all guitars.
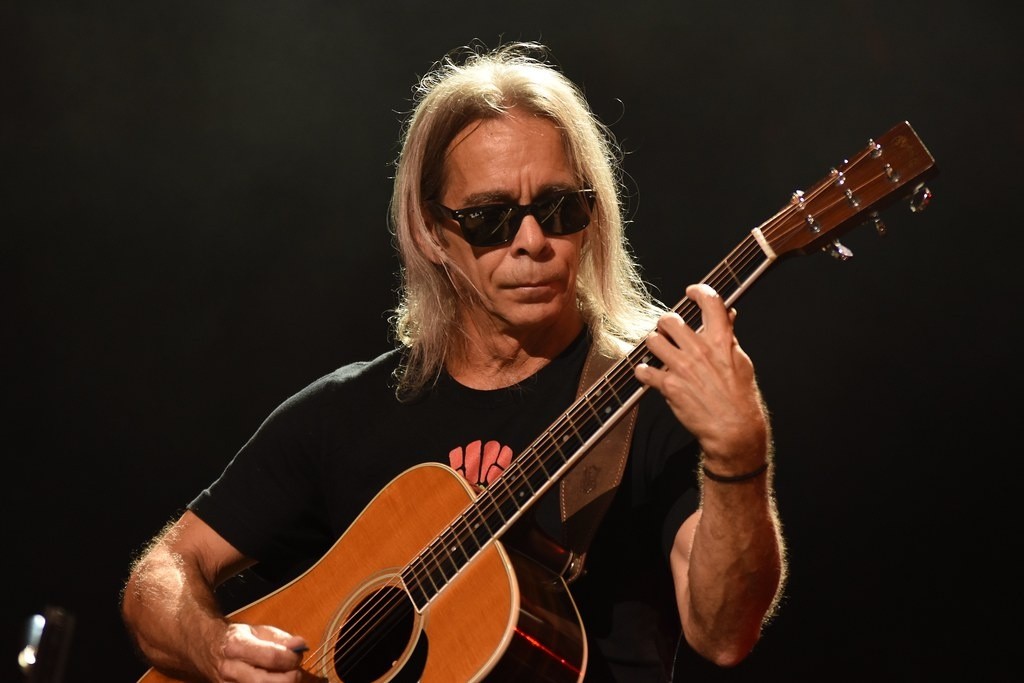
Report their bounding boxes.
[135,117,936,683]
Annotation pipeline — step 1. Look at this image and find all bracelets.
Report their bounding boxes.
[701,463,770,482]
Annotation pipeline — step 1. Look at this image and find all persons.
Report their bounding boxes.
[118,42,789,682]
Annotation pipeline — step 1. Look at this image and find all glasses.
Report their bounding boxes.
[433,186,597,247]
